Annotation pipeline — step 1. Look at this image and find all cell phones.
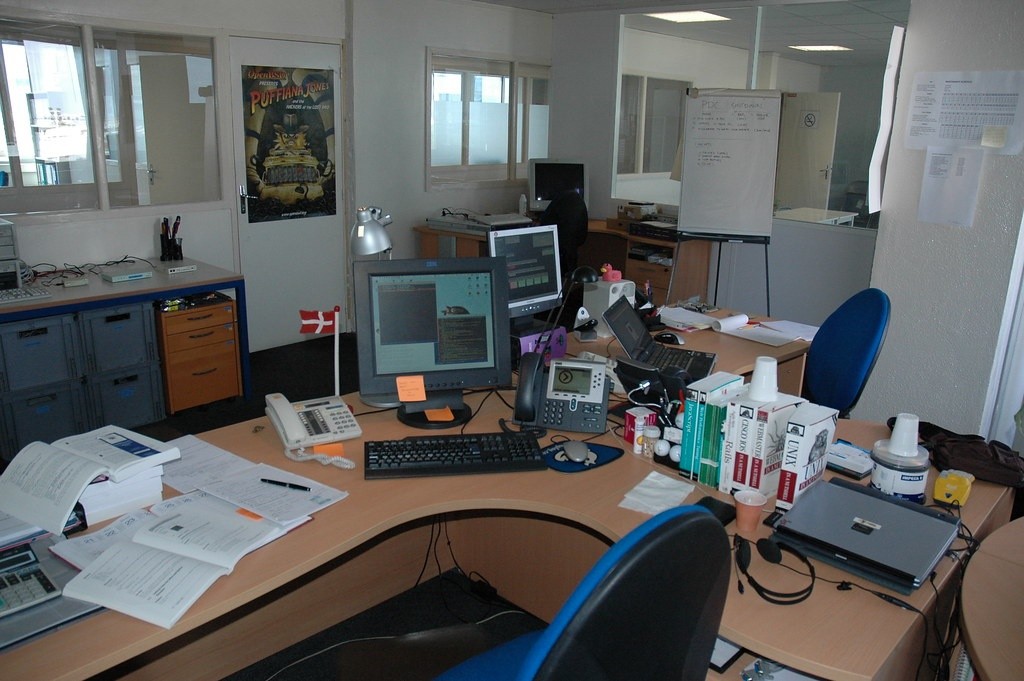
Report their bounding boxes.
[694,496,737,526]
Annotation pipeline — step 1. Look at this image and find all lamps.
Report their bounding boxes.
[534,266,597,352]
[348,205,393,261]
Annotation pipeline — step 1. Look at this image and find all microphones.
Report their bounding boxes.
[734,541,744,594]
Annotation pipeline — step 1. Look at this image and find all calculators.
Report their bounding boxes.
[0,543,61,620]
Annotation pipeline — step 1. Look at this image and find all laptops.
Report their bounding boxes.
[776,475,961,590]
[602,294,716,382]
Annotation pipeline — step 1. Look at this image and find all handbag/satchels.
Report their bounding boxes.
[925,432,1024,488]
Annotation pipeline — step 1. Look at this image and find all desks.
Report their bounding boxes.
[412,221,710,310]
[772,207,860,228]
[960,517,1024,681]
[0,302,1013,680]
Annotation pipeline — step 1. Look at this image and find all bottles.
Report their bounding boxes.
[633,418,661,459]
[518,194,526,216]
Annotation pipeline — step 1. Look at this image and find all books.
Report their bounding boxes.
[0,424,180,552]
[678,371,840,515]
[50,491,314,629]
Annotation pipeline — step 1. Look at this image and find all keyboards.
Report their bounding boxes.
[364,431,548,480]
[0,287,53,304]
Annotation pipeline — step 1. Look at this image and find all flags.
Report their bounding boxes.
[299,309,335,334]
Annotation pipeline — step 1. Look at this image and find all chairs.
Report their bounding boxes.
[836,179,876,229]
[800,287,891,418]
[428,505,732,681]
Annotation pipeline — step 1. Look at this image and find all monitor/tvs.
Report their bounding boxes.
[487,224,563,338]
[353,256,514,429]
[528,157,589,213]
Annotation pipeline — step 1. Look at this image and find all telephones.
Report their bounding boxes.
[265,393,362,451]
[514,351,612,433]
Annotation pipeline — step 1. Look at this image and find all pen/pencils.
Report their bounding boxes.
[172,216,180,238]
[162,218,168,238]
[665,416,672,426]
[660,398,664,409]
[262,479,310,491]
[664,389,671,403]
[643,280,652,296]
[748,321,760,323]
[658,416,665,424]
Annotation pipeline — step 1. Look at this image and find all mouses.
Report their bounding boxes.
[563,439,589,462]
[654,331,685,345]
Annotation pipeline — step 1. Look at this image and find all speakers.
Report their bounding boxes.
[583,279,635,338]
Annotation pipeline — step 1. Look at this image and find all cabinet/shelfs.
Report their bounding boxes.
[0,252,251,465]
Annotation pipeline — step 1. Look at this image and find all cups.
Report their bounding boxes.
[733,490,768,532]
[886,412,919,458]
[747,356,778,403]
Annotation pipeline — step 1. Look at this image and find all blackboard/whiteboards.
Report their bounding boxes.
[677,88,786,244]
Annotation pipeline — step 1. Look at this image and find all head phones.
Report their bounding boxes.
[733,533,815,605]
